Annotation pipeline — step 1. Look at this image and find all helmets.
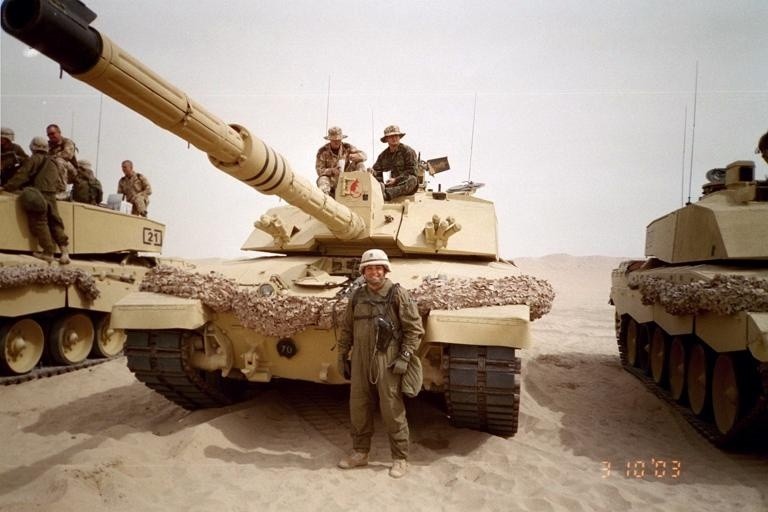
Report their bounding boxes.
[31,137,50,153]
[1,127,16,142]
[359,249,391,277]
[77,159,92,168]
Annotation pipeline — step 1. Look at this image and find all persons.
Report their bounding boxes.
[758,133,768,162]
[373,125,420,201]
[315,126,368,199]
[1,122,104,265]
[336,249,426,478]
[117,160,153,217]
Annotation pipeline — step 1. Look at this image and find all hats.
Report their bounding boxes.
[323,127,348,140]
[381,126,406,143]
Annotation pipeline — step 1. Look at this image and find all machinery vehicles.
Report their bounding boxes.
[608,158,767,459]
[0,188,165,386]
[1,2,554,438]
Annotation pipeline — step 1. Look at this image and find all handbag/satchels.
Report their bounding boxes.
[400,356,423,396]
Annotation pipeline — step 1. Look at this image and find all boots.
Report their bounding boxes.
[57,246,71,264]
[33,251,54,262]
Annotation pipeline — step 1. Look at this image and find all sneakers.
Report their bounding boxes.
[390,459,407,478]
[57,192,70,200]
[339,450,369,469]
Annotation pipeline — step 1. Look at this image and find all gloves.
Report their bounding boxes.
[335,359,350,380]
[390,353,410,375]
